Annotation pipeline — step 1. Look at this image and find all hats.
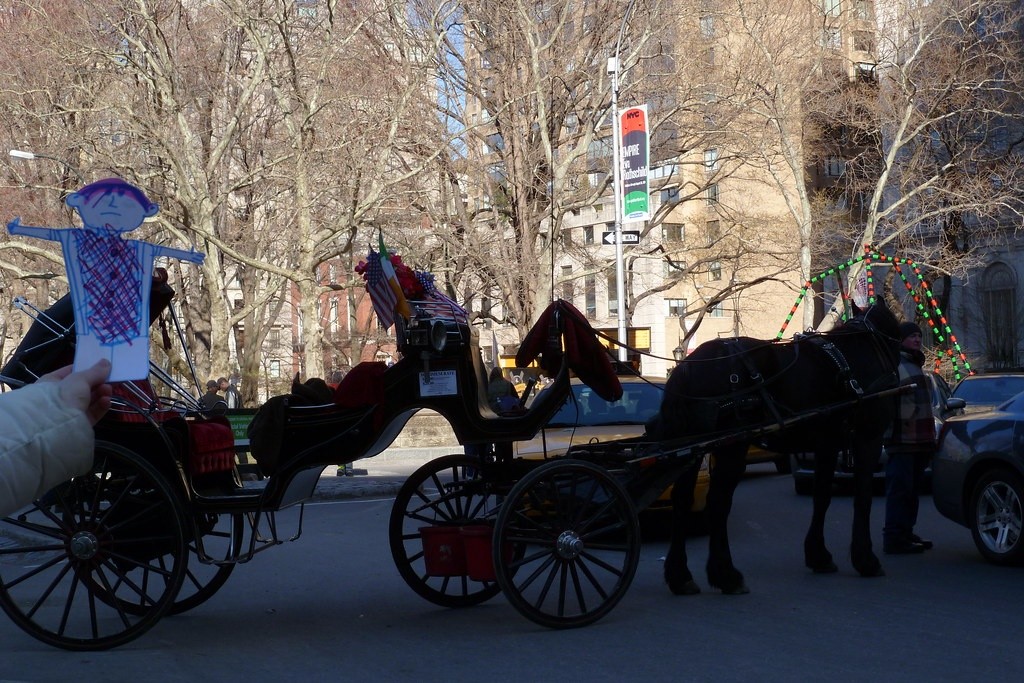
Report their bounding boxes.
[899,322,922,342]
[206,380,218,387]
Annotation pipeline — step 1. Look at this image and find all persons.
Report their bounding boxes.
[881,322,938,555]
[0,357,112,520]
[194,367,521,477]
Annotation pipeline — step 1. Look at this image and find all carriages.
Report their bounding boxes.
[0,245,915,651]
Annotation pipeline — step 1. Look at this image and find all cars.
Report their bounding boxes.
[792,366,1024,566]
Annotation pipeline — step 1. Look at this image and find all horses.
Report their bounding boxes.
[641,293,903,593]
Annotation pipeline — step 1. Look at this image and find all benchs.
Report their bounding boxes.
[369,254,480,344]
[117,379,237,475]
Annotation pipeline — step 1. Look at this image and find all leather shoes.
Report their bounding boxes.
[884,536,932,554]
